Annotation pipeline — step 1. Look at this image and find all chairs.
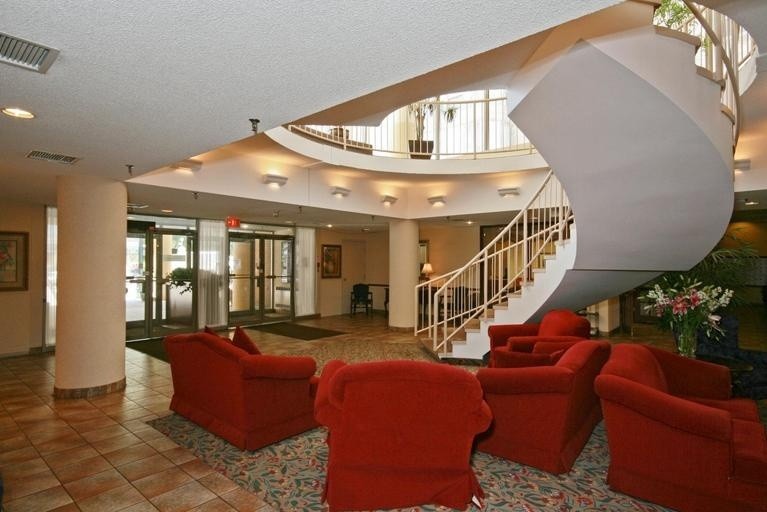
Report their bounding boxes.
[438,286,468,325]
[350,284,373,318]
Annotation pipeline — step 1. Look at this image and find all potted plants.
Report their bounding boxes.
[408,96,461,159]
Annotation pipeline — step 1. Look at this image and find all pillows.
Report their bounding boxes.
[233,327,260,354]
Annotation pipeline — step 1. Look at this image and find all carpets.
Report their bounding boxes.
[230,315,346,341]
[144,411,641,512]
[126,325,181,364]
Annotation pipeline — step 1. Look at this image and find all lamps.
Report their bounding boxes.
[422,263,433,279]
[382,195,398,207]
[171,158,201,172]
[427,196,447,207]
[332,186,350,198]
[498,187,520,198]
[262,174,287,188]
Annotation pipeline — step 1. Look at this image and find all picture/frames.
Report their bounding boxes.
[1,231,29,291]
[321,244,341,279]
[420,240,430,280]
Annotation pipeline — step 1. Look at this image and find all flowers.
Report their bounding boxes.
[635,274,734,359]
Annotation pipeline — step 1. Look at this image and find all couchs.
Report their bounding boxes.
[315,358,493,511]
[166,333,320,452]
[593,343,766,511]
[476,339,609,475]
[488,309,590,352]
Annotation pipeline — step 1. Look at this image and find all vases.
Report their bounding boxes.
[676,340,697,358]
